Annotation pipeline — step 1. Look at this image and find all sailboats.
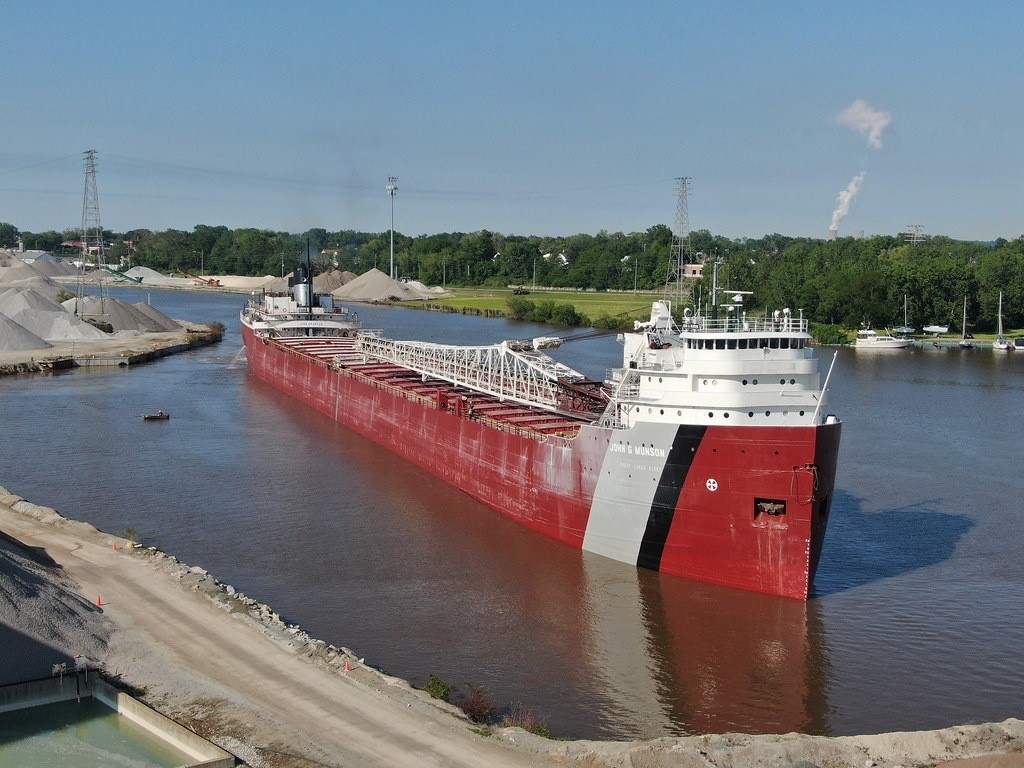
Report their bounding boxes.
[892,293,916,334]
[958,294,977,347]
[993,290,1010,349]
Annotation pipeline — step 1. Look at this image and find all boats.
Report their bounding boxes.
[1012,338,1024,349]
[239,239,843,602]
[922,324,950,334]
[142,409,169,421]
[853,314,913,349]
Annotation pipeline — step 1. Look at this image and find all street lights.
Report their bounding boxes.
[385,175,399,280]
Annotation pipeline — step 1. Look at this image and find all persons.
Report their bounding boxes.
[649,339,658,349]
